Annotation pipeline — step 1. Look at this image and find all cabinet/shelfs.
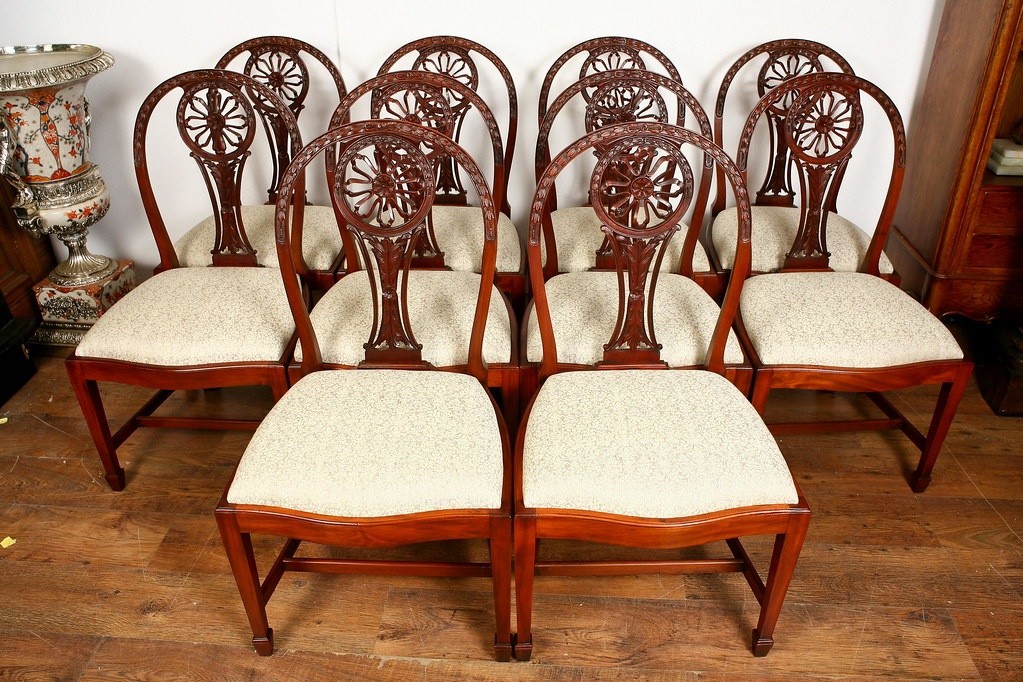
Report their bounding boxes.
[0,173,58,338]
[938,171,1023,326]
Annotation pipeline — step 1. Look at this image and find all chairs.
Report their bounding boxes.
[337,35,525,319]
[511,120,816,665]
[213,120,516,664]
[538,36,724,300]
[703,40,901,296]
[720,70,976,494]
[63,67,310,493]
[516,70,755,401]
[286,70,523,437]
[156,34,349,299]
